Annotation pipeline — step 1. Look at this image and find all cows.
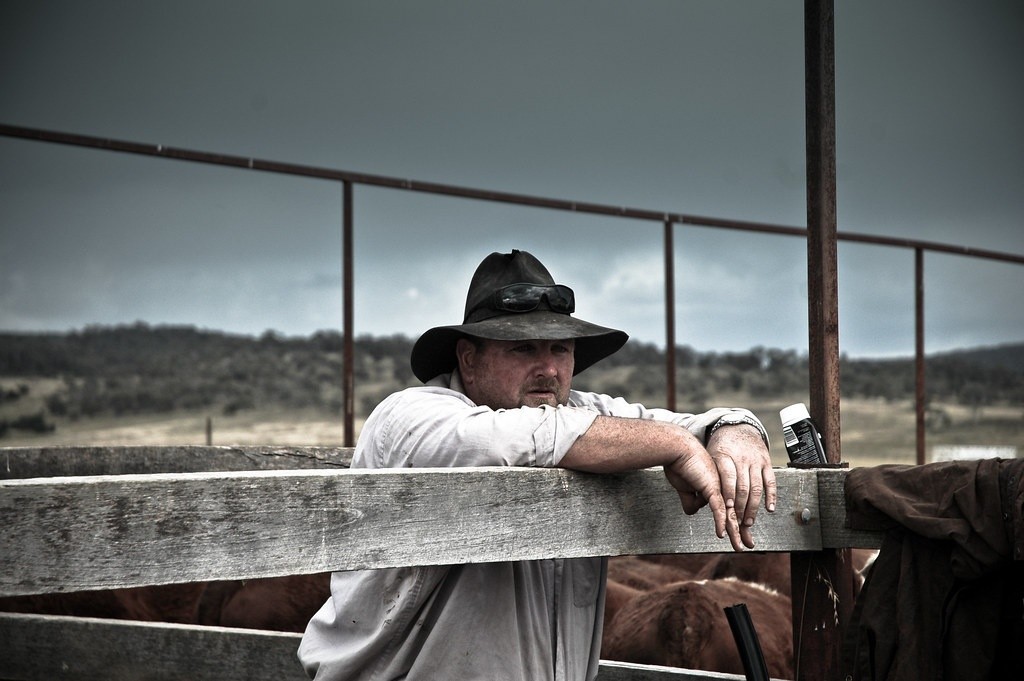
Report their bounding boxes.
[0,572,343,641]
[600,550,880,681]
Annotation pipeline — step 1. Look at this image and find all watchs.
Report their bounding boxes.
[710,413,766,440]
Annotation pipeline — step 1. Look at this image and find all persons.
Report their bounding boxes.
[297,249,778,681]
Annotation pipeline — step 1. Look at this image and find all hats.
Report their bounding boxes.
[411,249,630,385]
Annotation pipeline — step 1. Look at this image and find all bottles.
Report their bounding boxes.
[780,402,828,465]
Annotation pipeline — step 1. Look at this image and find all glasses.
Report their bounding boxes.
[463,282,575,325]
